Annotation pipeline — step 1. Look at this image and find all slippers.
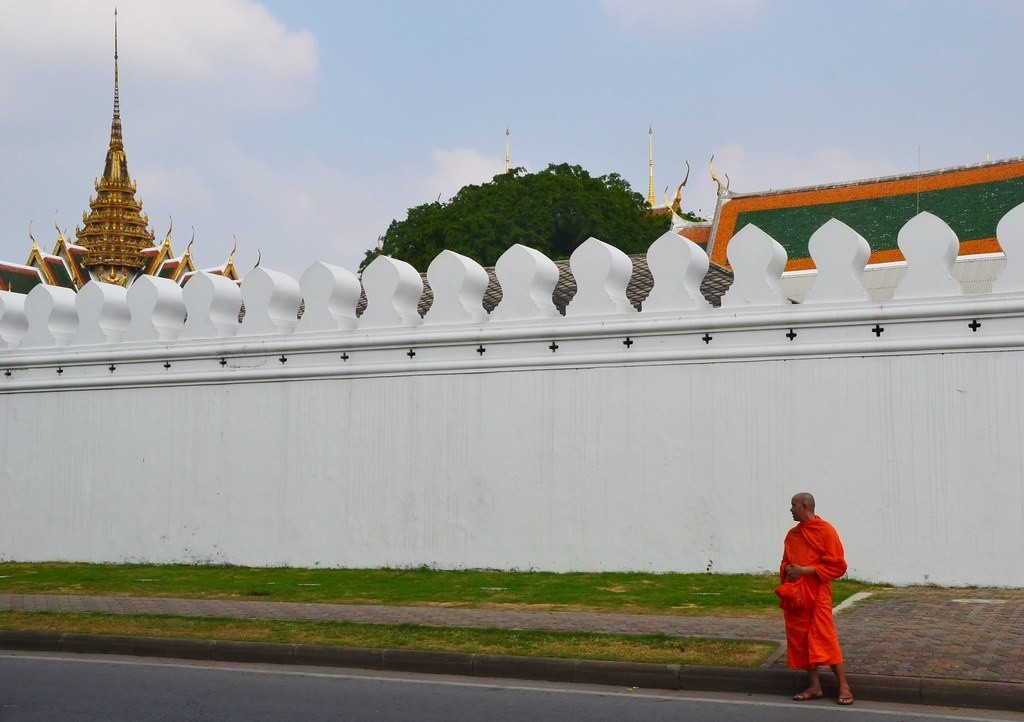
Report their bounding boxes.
[793,689,823,701]
[838,691,854,705]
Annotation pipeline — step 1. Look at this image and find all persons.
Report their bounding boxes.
[773,493,854,705]
